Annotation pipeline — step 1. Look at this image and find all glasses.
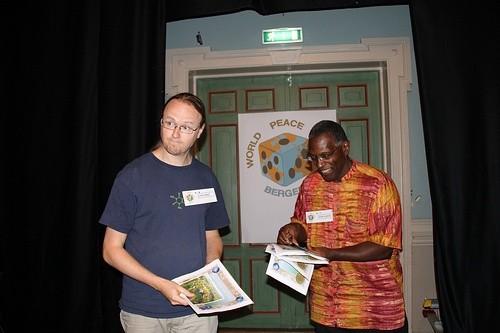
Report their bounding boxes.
[306,146,339,162]
[160,118,200,134]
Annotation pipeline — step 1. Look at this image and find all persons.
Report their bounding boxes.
[97,92,230,333]
[277,120,409,332]
